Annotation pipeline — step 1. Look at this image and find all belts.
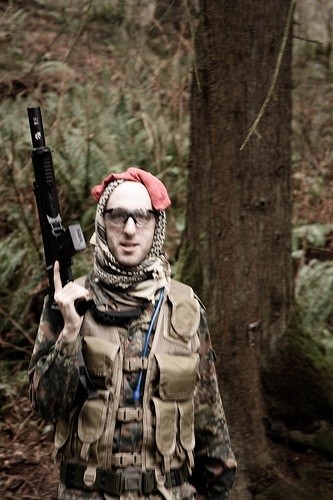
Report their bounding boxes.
[59,458,190,497]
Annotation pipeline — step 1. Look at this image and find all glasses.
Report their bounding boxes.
[104,207,157,226]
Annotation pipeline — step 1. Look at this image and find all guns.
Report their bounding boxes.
[26,103,88,325]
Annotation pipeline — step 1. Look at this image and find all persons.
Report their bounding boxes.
[27,164,238,499]
